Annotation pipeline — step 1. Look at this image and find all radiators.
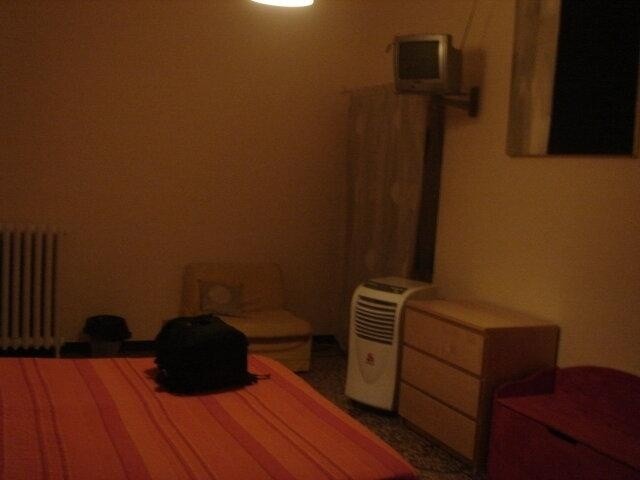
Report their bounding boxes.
[2,222,64,360]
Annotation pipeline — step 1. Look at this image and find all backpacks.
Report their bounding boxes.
[154,314,248,384]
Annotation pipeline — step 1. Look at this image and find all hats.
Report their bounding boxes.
[83,315,132,342]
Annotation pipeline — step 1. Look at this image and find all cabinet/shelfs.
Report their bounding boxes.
[397,300,559,469]
[487,364,640,479]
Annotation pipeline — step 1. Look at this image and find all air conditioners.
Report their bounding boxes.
[345,278,438,417]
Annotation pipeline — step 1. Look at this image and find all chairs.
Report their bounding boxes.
[180,263,312,375]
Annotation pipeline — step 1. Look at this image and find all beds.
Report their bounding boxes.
[0,352,417,480]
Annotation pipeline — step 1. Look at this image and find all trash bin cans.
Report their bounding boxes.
[84,316,132,354]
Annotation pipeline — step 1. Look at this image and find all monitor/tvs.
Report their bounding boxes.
[393,33,461,95]
